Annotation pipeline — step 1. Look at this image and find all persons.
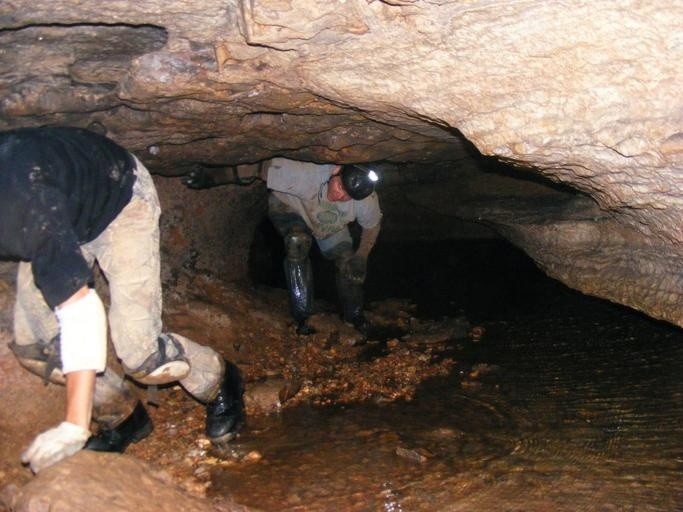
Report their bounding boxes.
[181,156,384,335]
[1,124,246,477]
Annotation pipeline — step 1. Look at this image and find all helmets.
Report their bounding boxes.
[341,164,384,200]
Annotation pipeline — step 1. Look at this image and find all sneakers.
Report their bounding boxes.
[297,322,315,334]
[85,400,154,451]
[345,315,377,340]
[206,360,245,445]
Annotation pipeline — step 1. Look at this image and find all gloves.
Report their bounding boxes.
[22,422,90,474]
[341,256,367,285]
[184,168,221,188]
